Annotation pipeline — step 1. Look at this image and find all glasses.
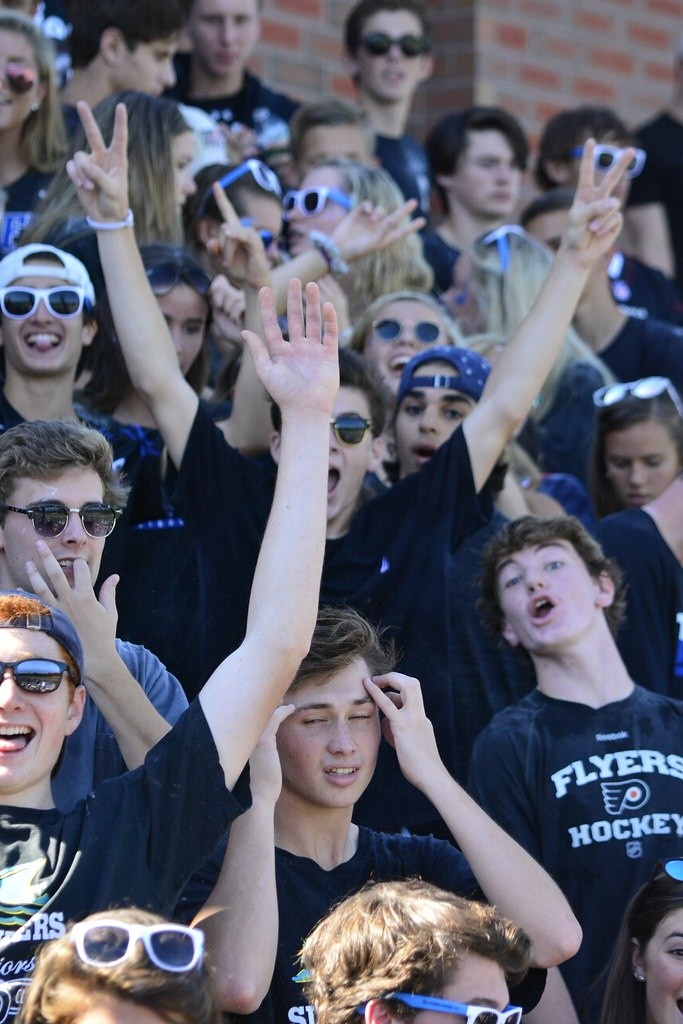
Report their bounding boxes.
[566,144,645,178]
[355,992,523,1024]
[372,319,441,343]
[260,229,275,249]
[148,264,214,298]
[0,285,85,319]
[0,68,43,93]
[0,657,76,693]
[5,497,116,539]
[650,857,683,883]
[63,920,206,973]
[283,189,350,217]
[332,413,376,445]
[591,376,683,422]
[196,159,286,217]
[359,32,424,57]
[479,224,526,283]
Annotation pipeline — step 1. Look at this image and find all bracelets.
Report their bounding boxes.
[312,246,334,268]
[84,209,133,232]
[308,228,350,276]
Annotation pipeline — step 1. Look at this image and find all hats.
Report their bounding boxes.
[0,589,83,687]
[0,243,95,314]
[395,345,490,400]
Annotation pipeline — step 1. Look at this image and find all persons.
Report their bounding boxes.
[0,0,683,1024]
